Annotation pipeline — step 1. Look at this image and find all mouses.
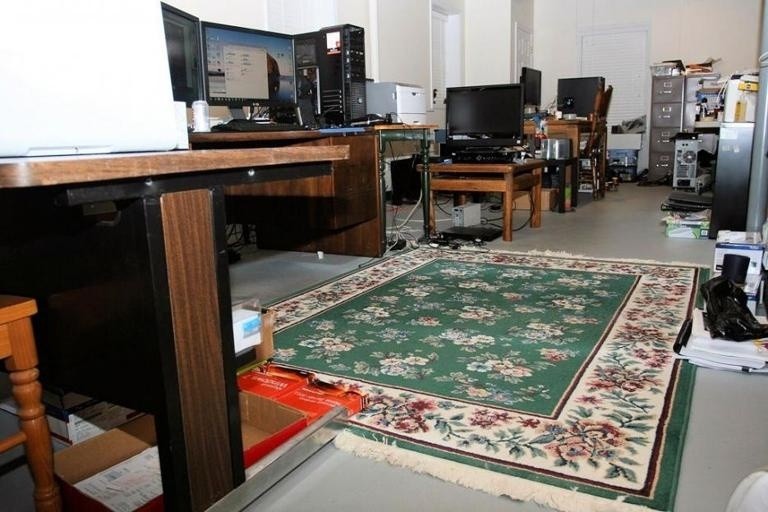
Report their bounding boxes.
[303,125,312,130]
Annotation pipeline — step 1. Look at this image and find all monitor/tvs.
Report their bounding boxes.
[520,66,542,119]
[160,2,203,133]
[445,83,525,153]
[200,21,297,125]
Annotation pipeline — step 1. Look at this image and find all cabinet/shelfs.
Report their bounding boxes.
[648,76,686,185]
[694,86,727,135]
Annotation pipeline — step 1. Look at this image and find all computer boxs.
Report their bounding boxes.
[556,77,606,120]
[452,203,481,228]
[293,23,367,126]
[672,138,703,191]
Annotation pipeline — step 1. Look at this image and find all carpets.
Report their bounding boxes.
[254,238,712,510]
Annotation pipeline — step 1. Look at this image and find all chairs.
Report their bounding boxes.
[0,297,63,511]
[578,85,614,203]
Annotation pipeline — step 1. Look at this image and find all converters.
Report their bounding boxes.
[387,239,407,251]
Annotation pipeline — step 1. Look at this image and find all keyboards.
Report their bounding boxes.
[210,123,305,133]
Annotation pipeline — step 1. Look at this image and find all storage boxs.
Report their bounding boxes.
[715,230,765,316]
[501,187,559,212]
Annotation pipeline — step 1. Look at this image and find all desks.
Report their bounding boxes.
[188,127,384,258]
[1,144,352,510]
[522,119,608,213]
[415,158,545,243]
[363,123,438,244]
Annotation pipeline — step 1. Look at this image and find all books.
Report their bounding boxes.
[669,306,767,375]
[686,56,722,73]
